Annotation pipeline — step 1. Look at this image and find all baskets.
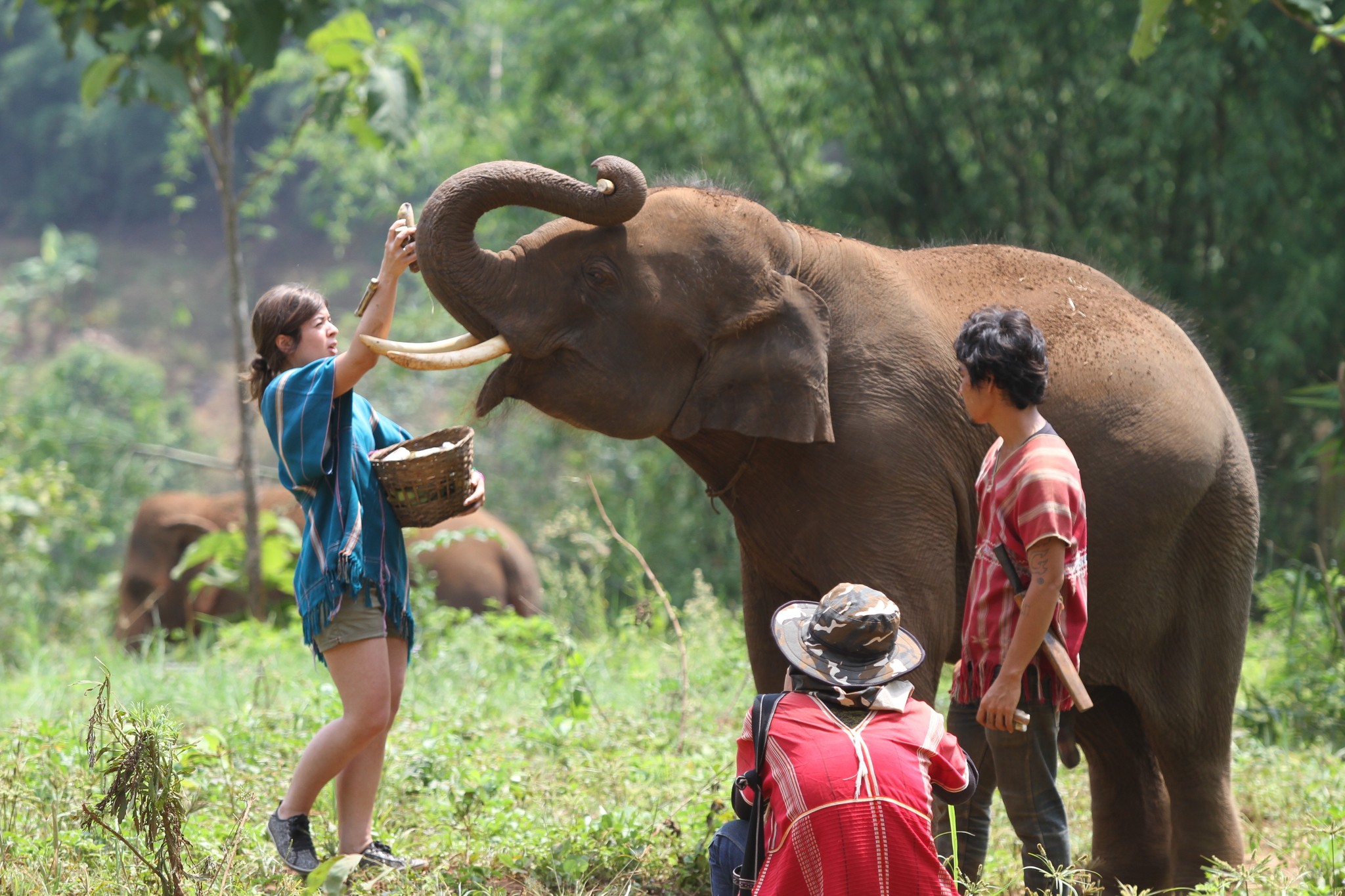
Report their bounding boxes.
[369,426,477,528]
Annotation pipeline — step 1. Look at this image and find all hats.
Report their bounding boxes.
[771,582,926,687]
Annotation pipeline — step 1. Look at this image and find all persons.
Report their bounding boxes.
[244,219,486,874]
[707,582,979,896]
[931,306,1089,895]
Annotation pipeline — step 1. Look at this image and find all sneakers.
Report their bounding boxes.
[267,801,319,874]
[358,841,426,878]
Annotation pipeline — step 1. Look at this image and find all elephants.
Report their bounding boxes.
[412,154,1261,896]
[117,485,540,652]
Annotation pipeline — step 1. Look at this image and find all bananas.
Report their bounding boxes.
[397,202,421,272]
[380,442,457,505]
